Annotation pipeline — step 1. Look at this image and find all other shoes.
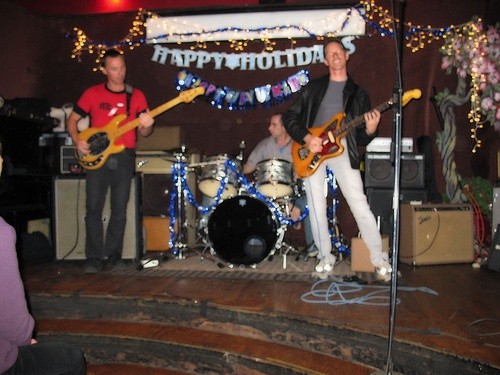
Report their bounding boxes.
[315,261,333,273]
[375,265,401,281]
[309,248,319,257]
[84,264,100,273]
[100,259,115,271]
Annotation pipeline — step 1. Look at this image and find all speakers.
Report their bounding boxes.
[366,187,426,249]
[54,172,142,259]
[364,152,423,190]
[399,201,476,267]
[141,213,187,251]
[140,172,172,210]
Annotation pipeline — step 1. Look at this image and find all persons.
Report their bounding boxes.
[66,48,155,274]
[0,142,86,375]
[242,113,319,258]
[283,36,402,282]
[417,136,436,201]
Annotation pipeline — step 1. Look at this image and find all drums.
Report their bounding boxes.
[255,158,295,199]
[198,156,239,199]
[209,196,278,266]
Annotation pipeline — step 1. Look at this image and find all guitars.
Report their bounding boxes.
[74,86,206,170]
[291,88,422,177]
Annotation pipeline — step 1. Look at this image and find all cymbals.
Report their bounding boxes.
[164,157,190,163]
